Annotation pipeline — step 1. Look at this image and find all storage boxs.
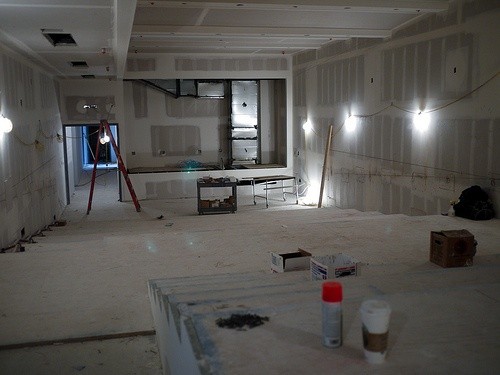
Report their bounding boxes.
[310,254,359,282]
[429,229,478,267]
[269,247,313,272]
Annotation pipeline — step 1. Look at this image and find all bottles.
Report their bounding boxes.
[359,300,392,364]
[321,281,344,347]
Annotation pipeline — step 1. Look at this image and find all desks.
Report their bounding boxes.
[242,175,298,208]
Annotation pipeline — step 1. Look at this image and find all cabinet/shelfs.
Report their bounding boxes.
[196,175,237,214]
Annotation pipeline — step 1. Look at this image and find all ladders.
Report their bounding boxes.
[86,119,140,214]
